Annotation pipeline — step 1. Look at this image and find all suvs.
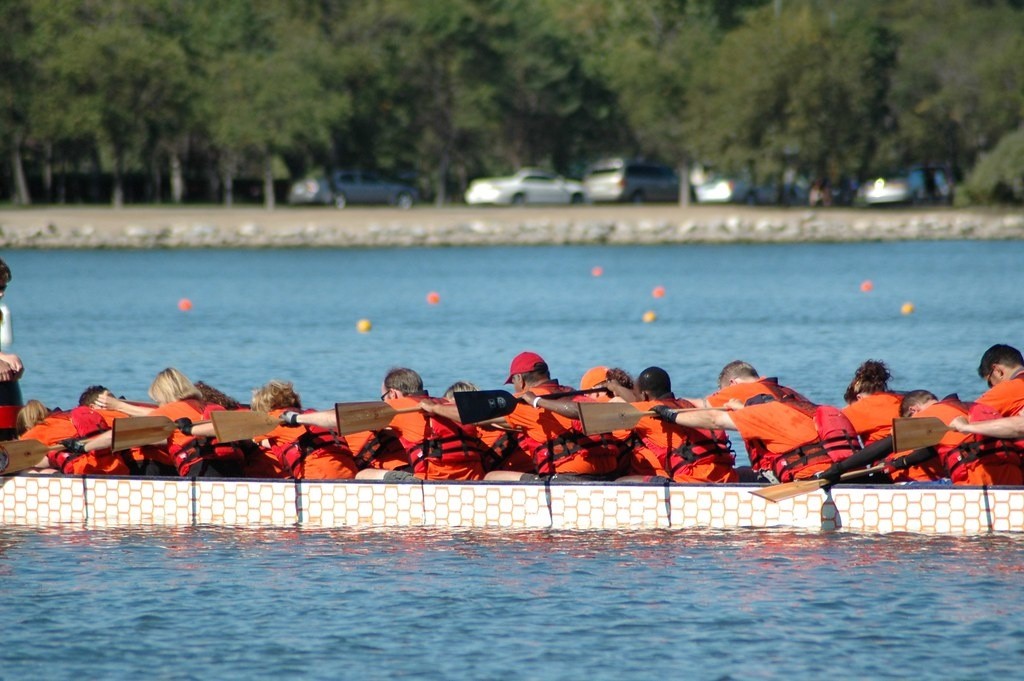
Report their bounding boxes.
[583,155,678,206]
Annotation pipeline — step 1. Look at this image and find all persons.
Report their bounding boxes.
[947,343,1024,486]
[416,350,836,483]
[0,259,37,382]
[16,398,130,475]
[838,357,943,483]
[58,367,414,481]
[813,390,972,492]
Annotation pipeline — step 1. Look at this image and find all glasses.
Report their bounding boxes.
[380,390,390,401]
[988,369,994,387]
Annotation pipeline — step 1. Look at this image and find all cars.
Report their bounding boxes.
[290,166,422,213]
[460,166,584,207]
[691,169,785,203]
[861,169,954,206]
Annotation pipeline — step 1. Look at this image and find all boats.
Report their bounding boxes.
[0,467,1024,539]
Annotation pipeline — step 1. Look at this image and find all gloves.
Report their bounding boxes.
[59,438,89,453]
[647,404,678,424]
[175,416,193,436]
[278,410,301,427]
[818,468,836,491]
[880,454,908,474]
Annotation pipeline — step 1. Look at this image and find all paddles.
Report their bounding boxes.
[0,389,965,504]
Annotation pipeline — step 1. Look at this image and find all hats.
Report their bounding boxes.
[581,365,610,390]
[503,351,545,384]
[743,393,768,405]
